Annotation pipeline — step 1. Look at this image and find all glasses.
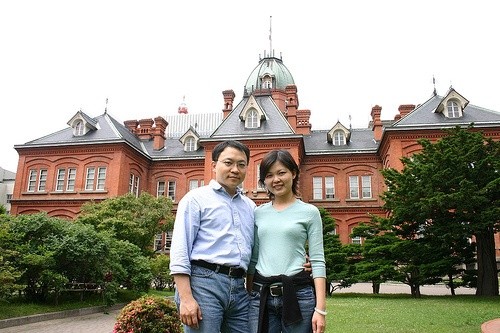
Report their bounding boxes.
[218,159,247,168]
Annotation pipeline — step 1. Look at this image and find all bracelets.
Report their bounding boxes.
[314,306,327,316]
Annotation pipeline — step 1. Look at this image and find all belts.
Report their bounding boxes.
[190,259,245,278]
[252,282,310,297]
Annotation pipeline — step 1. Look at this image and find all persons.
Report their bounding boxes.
[168,139,312,332]
[247,149,327,333]
